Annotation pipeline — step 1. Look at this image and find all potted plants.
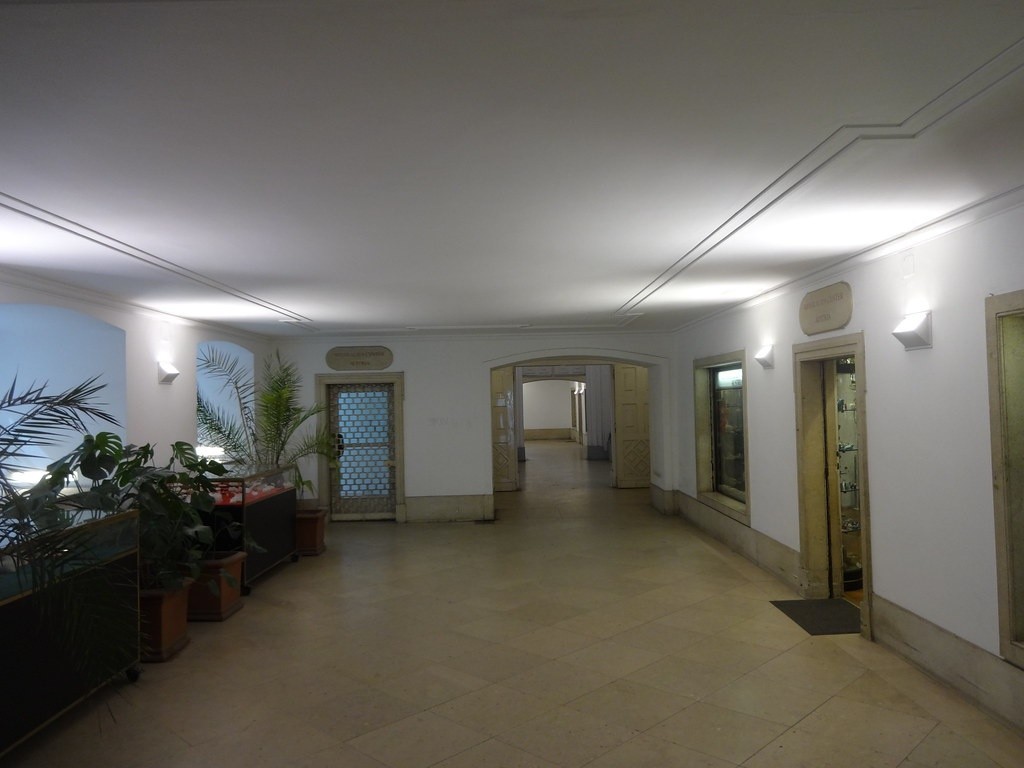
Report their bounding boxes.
[48,355,345,665]
